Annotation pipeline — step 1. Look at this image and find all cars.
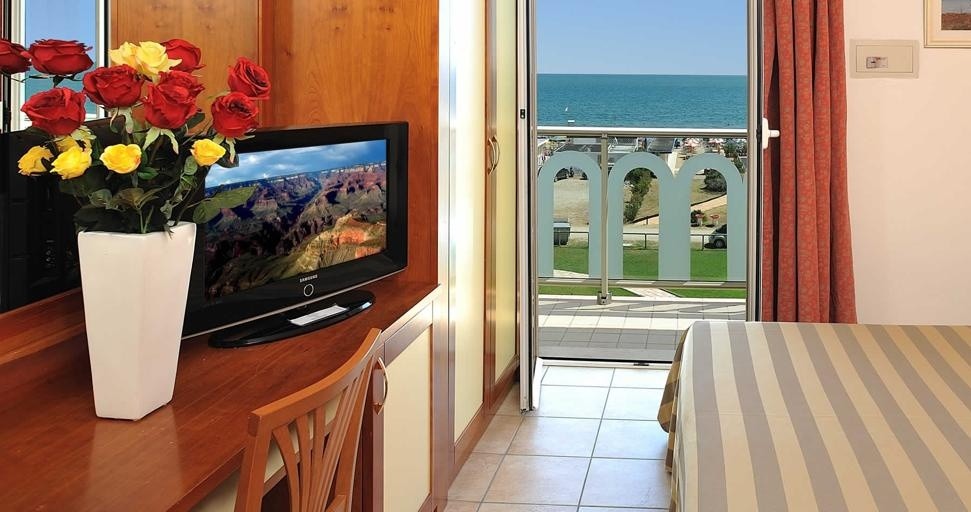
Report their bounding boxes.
[709,223,727,250]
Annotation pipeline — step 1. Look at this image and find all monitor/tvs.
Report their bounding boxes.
[0,115,126,316]
[180,121,410,350]
